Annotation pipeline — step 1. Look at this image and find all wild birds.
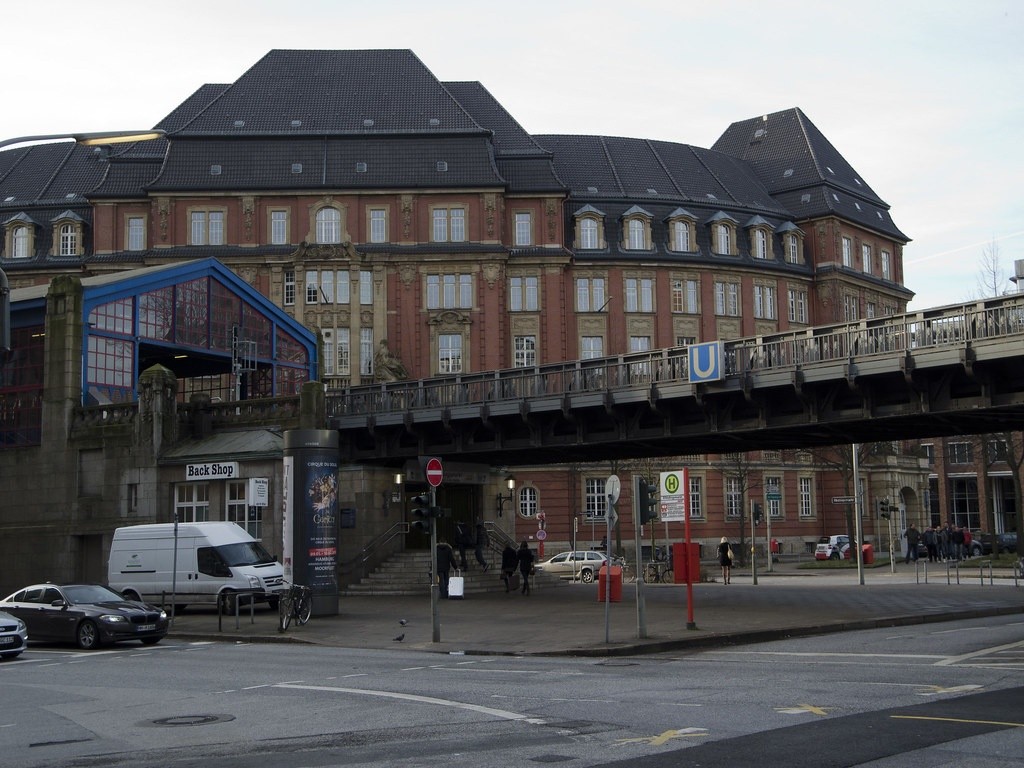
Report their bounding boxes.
[392,619,411,642]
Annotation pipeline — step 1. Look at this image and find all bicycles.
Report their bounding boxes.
[280,579,312,630]
[643,553,673,584]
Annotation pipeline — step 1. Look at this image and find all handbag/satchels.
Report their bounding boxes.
[429,571,439,586]
[500,570,505,579]
[728,544,734,560]
[509,570,520,589]
[462,535,472,547]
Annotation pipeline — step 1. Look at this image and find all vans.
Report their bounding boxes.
[108,521,283,616]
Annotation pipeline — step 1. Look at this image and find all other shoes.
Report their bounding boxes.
[522,587,526,593]
[728,582,730,584]
[506,590,510,592]
[724,582,726,585]
[527,588,530,596]
[483,564,489,572]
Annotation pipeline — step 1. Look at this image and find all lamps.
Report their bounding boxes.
[382,468,406,509]
[496,475,517,517]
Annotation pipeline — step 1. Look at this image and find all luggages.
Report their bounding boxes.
[448,568,464,600]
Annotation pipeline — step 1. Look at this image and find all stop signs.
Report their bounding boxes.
[427,459,443,487]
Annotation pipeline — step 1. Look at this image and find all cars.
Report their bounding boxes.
[815,534,857,561]
[0,610,29,661]
[533,550,622,583]
[980,533,1017,555]
[0,582,169,651]
[918,539,984,557]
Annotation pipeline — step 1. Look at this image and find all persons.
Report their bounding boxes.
[472,520,490,572]
[903,524,920,564]
[716,536,732,585]
[517,541,533,596]
[455,520,467,566]
[922,521,971,563]
[501,543,517,593]
[436,536,458,599]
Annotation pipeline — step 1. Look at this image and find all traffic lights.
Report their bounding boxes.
[640,484,658,525]
[880,499,890,520]
[755,504,763,520]
[411,494,429,531]
